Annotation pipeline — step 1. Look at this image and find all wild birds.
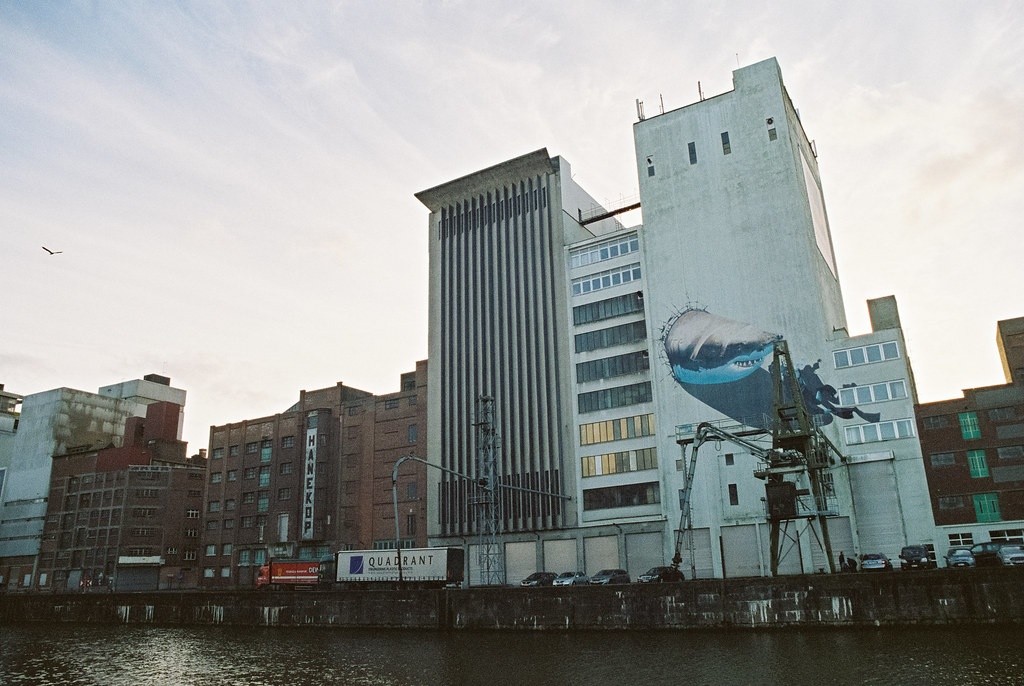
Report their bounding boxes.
[41,245,64,258]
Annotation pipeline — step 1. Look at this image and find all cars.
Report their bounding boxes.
[553,571,591,586]
[996,544,1024,566]
[520,570,559,586]
[943,548,978,568]
[636,566,685,583]
[861,551,893,570]
[587,569,630,584]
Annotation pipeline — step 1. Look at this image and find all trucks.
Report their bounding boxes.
[255,557,329,590]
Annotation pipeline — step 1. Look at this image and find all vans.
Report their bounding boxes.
[970,541,1024,565]
[898,544,934,570]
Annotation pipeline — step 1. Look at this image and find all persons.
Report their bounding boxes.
[847,558,858,573]
[839,551,846,571]
